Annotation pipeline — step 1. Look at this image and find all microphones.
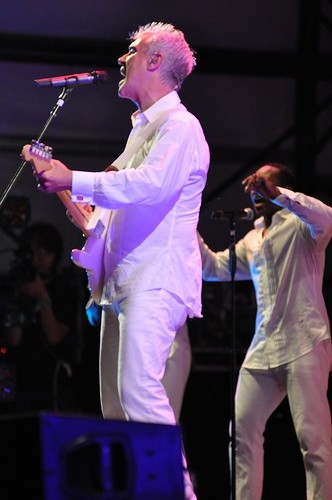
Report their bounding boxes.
[211,207,254,221]
[33,70,108,87]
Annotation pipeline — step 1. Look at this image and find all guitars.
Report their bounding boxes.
[19,140,112,328]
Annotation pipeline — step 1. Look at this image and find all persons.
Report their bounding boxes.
[33,21,210,500]
[196,164,332,500]
[10,221,90,414]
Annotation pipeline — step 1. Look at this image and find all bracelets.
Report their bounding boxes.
[34,300,52,312]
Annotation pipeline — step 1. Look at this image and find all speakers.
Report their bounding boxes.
[0,409,185,500]
